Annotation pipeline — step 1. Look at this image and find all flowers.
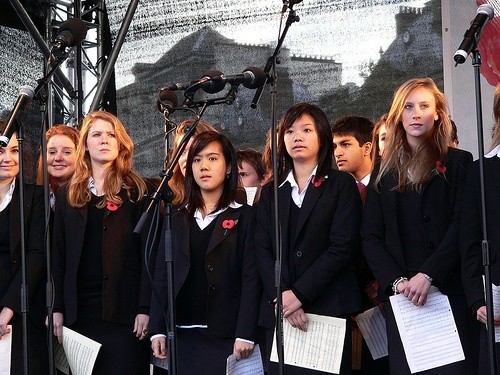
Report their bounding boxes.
[105,201,119,217]
[222,219,239,235]
[427,160,447,185]
[310,173,329,192]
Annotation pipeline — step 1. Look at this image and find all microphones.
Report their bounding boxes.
[157,89,179,117]
[282,0,303,6]
[453,4,494,67]
[186,70,226,94]
[0,84,35,148]
[212,67,266,90]
[51,19,87,54]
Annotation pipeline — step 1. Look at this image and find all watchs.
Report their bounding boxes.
[424,274,432,281]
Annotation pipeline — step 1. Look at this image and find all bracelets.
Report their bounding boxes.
[392,277,407,294]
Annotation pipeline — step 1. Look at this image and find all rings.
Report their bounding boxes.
[142,329,148,335]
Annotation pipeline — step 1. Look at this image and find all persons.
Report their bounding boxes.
[0,120,51,375]
[148,131,265,375]
[475,83,500,375]
[254,103,362,375]
[361,78,473,375]
[44,111,159,375]
[34,115,459,375]
